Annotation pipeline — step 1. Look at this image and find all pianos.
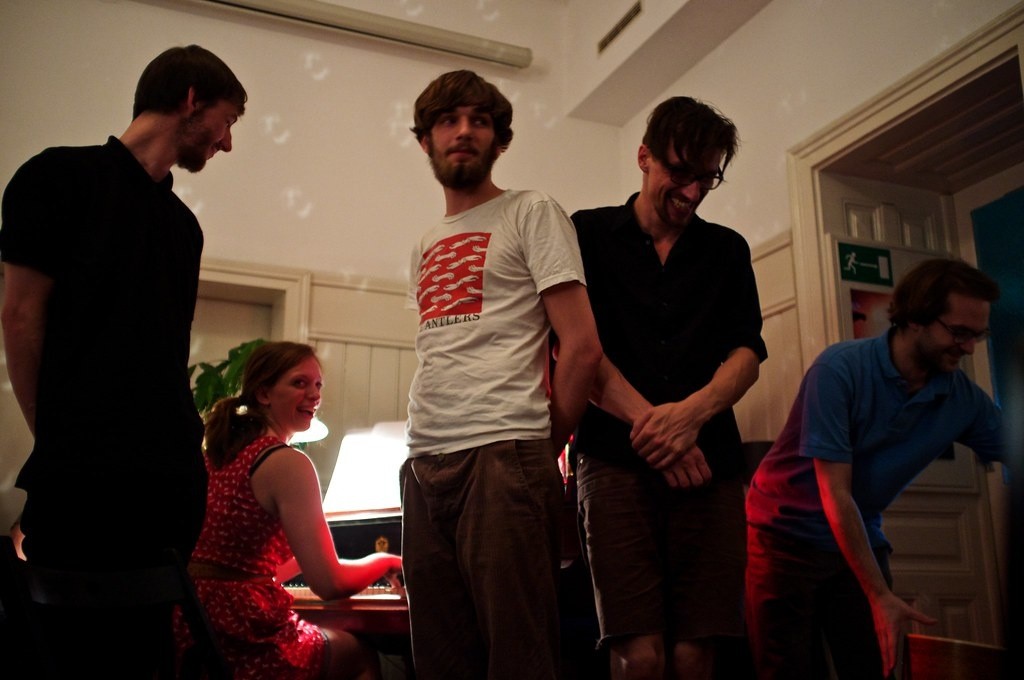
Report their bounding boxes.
[276,506,413,680]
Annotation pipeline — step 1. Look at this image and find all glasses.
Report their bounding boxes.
[659,155,723,190]
[936,316,993,343]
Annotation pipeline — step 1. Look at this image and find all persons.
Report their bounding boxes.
[548,94,769,680]
[745,257,1024,680]
[174,341,405,680]
[1,44,248,680]
[397,70,606,680]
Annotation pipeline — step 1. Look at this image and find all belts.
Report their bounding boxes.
[188,561,255,581]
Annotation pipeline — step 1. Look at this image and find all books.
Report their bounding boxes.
[319,430,405,518]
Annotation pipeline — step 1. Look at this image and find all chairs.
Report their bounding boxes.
[894,625,1005,680]
